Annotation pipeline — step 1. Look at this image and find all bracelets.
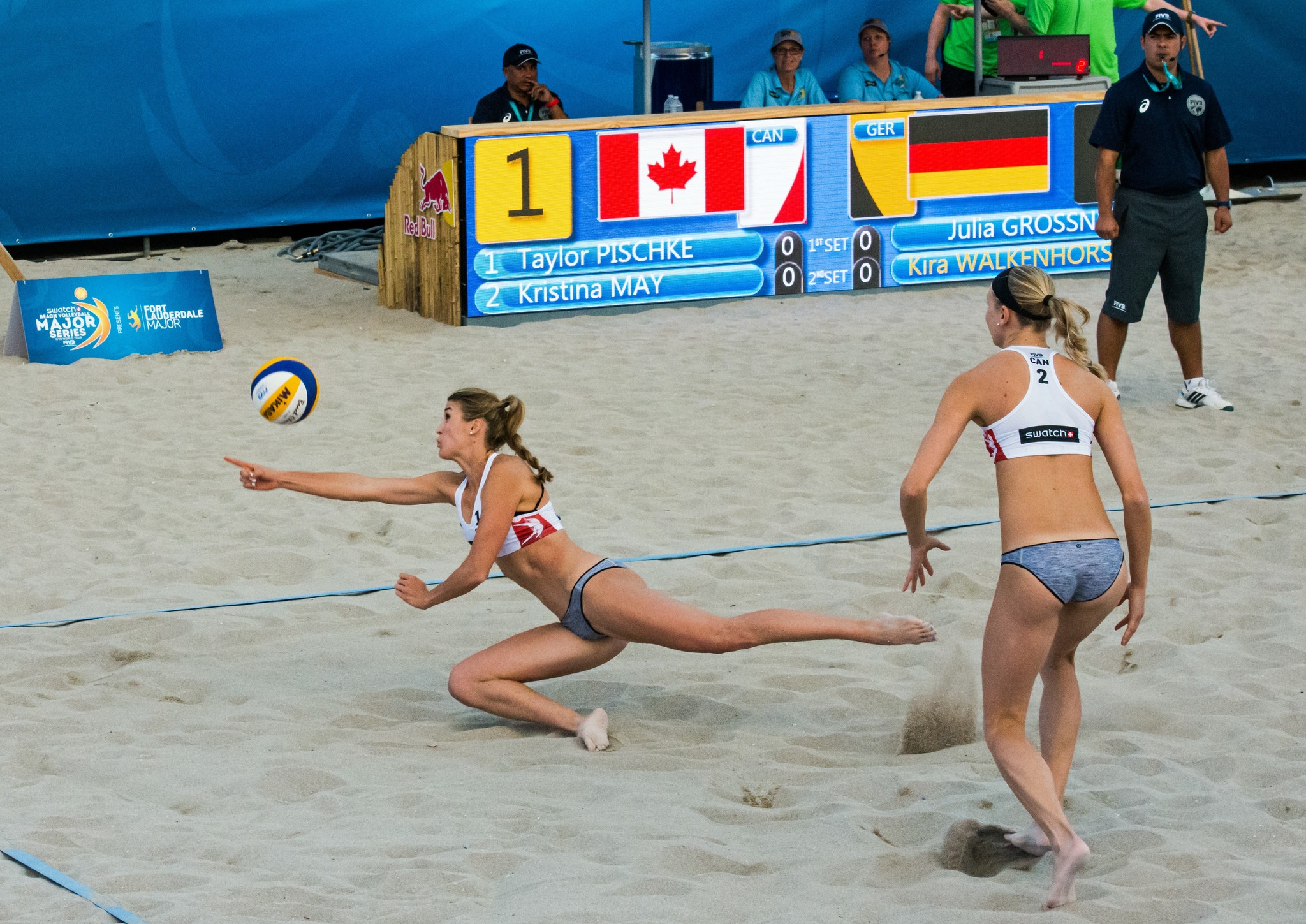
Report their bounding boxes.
[545,97,559,108]
[908,532,927,549]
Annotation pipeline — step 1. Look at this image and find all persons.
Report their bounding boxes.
[837,18,946,103]
[946,0,1228,86]
[1089,7,1235,412]
[225,388,938,752]
[925,0,1029,98]
[740,29,830,108]
[472,43,569,124]
[900,266,1150,908]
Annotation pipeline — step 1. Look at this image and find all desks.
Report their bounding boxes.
[378,90,1117,329]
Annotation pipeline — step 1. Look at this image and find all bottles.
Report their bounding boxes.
[671,96,683,113]
[913,91,923,100]
[664,94,673,113]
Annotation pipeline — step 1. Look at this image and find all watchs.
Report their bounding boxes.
[1187,10,1196,24]
[1215,200,1232,210]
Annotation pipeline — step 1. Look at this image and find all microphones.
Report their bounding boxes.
[1171,42,1184,62]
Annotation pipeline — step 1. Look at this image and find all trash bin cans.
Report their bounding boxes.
[635,42,714,114]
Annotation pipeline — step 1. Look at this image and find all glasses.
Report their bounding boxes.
[776,46,802,55]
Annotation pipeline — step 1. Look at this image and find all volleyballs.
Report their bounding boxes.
[251,356,318,425]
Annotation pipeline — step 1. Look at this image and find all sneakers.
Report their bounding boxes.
[1175,379,1234,412]
[1106,379,1120,401]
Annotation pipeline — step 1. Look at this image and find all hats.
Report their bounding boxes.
[858,19,889,34]
[503,43,541,67]
[1143,8,1182,35]
[771,28,803,48]
